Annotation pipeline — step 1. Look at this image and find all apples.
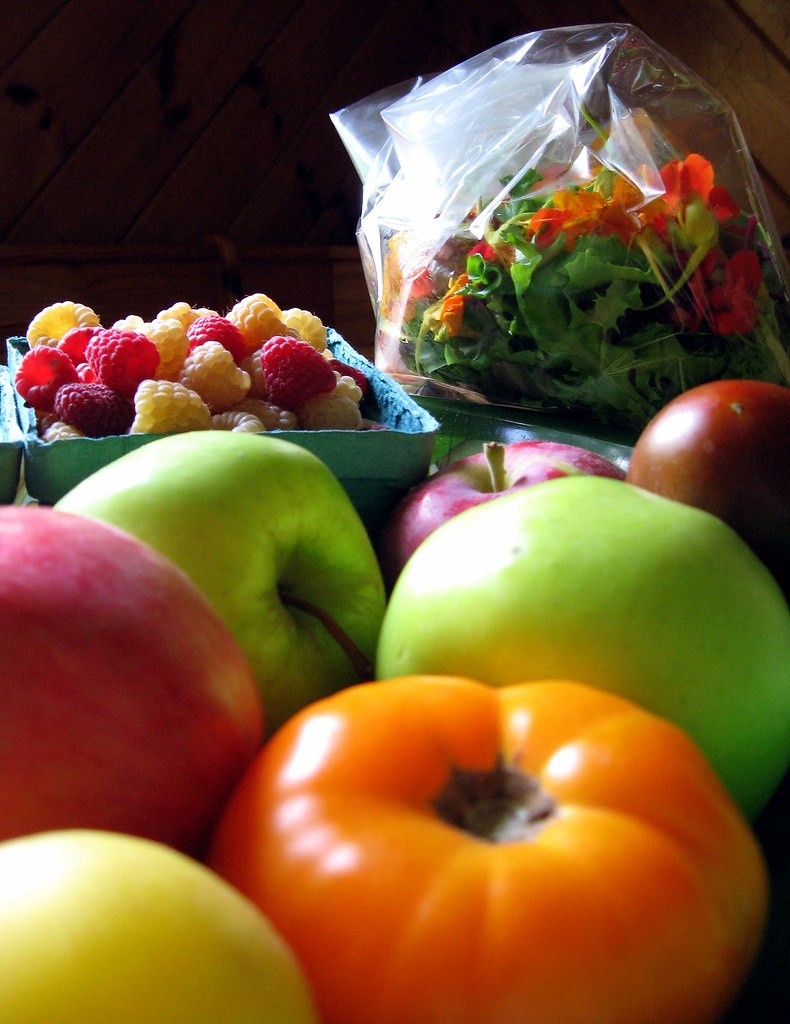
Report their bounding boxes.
[45,430,389,732]
[360,436,631,591]
[374,474,790,840]
[0,503,264,853]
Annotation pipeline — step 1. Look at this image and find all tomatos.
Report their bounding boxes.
[200,671,765,1024]
[0,826,316,1024]
[623,381,790,599]
